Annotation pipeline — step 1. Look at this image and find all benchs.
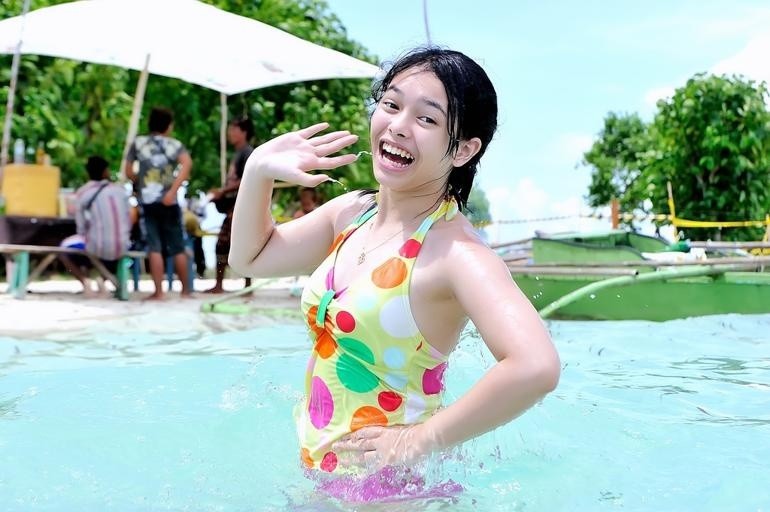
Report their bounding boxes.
[0,243,148,301]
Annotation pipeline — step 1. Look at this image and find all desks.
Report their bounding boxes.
[6,211,143,250]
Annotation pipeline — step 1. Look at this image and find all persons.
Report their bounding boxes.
[203,118,256,296]
[59,154,137,300]
[125,107,198,300]
[292,184,319,285]
[227,46,561,512]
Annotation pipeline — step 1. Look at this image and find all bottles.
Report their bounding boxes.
[14,139,44,165]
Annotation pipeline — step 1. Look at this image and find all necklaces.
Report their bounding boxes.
[357,219,417,267]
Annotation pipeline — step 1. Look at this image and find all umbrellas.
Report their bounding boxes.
[0,0,382,182]
